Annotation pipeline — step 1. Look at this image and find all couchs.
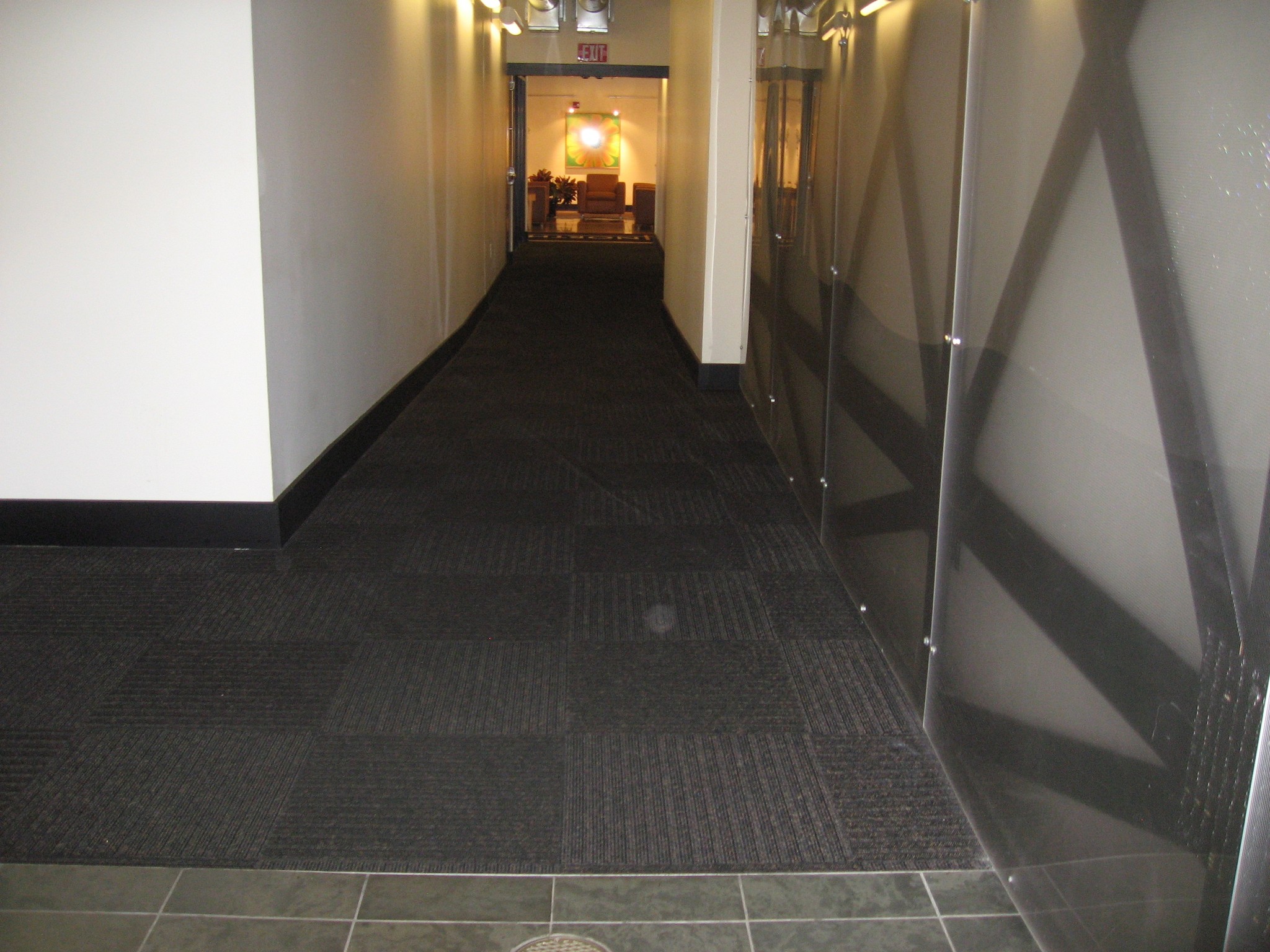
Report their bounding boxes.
[633,183,656,232]
[577,174,626,222]
[528,181,550,227]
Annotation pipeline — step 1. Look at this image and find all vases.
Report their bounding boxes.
[549,198,557,217]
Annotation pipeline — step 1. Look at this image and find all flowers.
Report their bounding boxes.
[528,168,577,206]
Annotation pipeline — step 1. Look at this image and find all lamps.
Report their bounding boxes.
[492,6,524,36]
